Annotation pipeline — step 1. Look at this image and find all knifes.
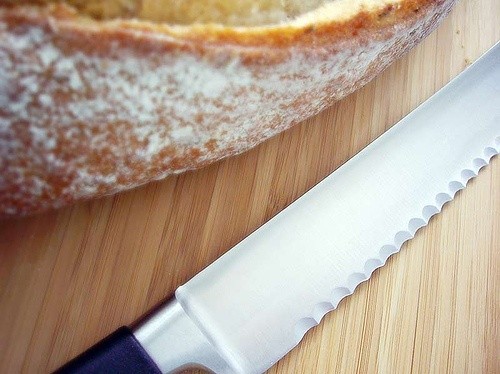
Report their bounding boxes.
[52,42,500,374]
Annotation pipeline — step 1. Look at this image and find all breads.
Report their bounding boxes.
[0,0,457,219]
